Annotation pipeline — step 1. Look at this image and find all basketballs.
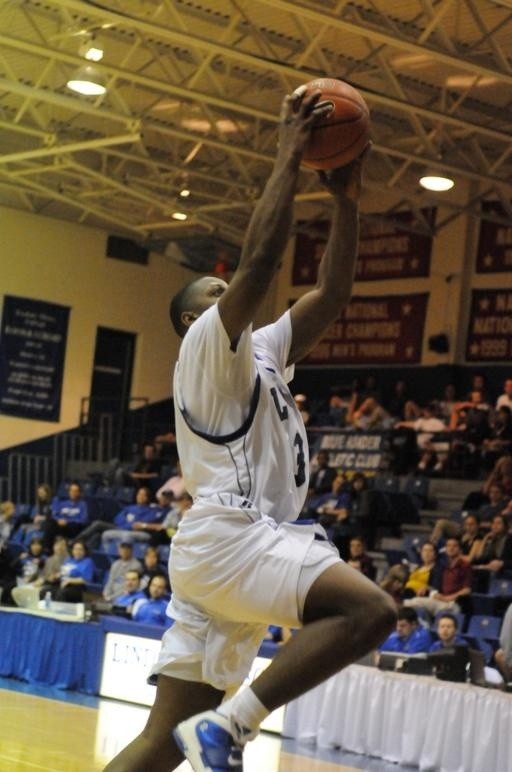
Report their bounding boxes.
[294,78,371,171]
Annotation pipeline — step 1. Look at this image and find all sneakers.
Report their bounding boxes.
[172,708,259,772]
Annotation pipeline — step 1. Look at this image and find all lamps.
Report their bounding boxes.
[418,146,455,192]
[63,63,107,98]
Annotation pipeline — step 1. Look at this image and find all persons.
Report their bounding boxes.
[262,372,512,691]
[0,432,193,627]
[102,85,399,770]
